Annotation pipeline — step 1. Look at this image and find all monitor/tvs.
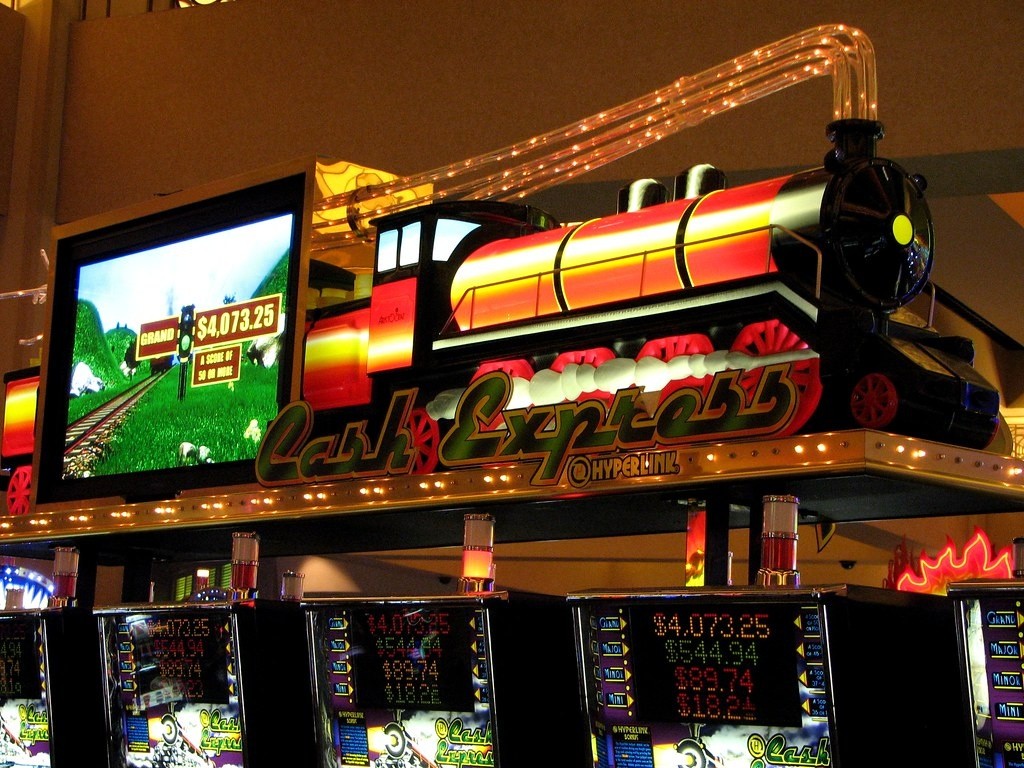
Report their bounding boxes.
[947,587,1024,768]
[570,593,839,768]
[28,152,308,507]
[92,606,249,768]
[303,599,500,768]
[0,609,56,768]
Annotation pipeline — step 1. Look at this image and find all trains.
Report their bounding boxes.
[1,23,1006,512]
